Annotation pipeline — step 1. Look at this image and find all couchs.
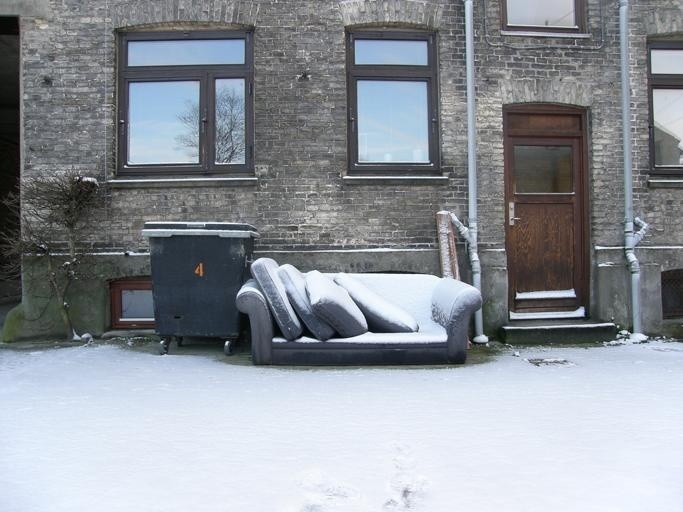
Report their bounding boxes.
[237,273,482,364]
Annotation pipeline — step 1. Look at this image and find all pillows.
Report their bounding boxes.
[250,257,303,340]
[278,263,334,340]
[334,271,419,332]
[304,269,368,337]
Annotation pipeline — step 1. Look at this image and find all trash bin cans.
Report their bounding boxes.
[140,220,260,355]
